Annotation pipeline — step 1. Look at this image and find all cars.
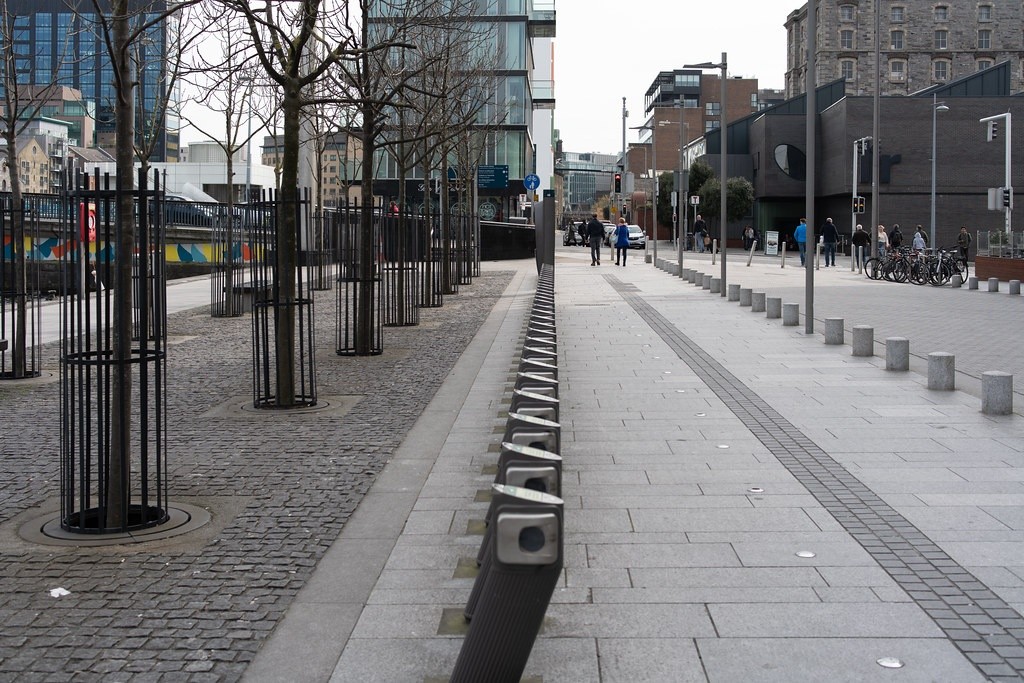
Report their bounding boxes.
[221,201,270,228]
[562,220,618,247]
[149,195,219,226]
[628,225,646,249]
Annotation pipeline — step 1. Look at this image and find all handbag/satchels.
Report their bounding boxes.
[700,228,707,237]
[609,226,620,244]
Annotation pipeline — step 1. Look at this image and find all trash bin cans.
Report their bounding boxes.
[687,233,693,250]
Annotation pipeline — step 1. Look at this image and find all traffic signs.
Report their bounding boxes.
[448,165,509,190]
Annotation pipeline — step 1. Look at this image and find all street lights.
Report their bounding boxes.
[240,76,252,204]
[659,121,688,250]
[931,101,951,254]
[683,62,727,297]
[629,125,658,267]
[628,147,646,231]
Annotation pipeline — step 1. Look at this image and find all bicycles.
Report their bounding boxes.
[864,245,968,287]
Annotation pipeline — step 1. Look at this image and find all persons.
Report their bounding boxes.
[911,225,928,264]
[614,218,629,266]
[585,213,605,266]
[741,224,759,251]
[851,224,871,269]
[888,224,903,260]
[783,234,796,251]
[957,226,972,267]
[578,219,587,247]
[793,218,807,266]
[388,200,400,212]
[566,219,577,246]
[693,215,706,253]
[819,217,840,267]
[878,225,889,259]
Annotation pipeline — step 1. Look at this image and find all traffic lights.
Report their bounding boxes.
[988,121,997,143]
[615,173,622,194]
[623,204,627,216]
[857,139,864,157]
[853,197,865,214]
[1003,188,1013,209]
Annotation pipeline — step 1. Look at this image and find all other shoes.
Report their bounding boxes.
[615,262,619,265]
[825,264,828,267]
[831,264,835,266]
[623,262,625,266]
[597,260,600,265]
[591,263,595,266]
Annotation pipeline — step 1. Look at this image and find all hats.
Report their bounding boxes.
[827,218,832,222]
